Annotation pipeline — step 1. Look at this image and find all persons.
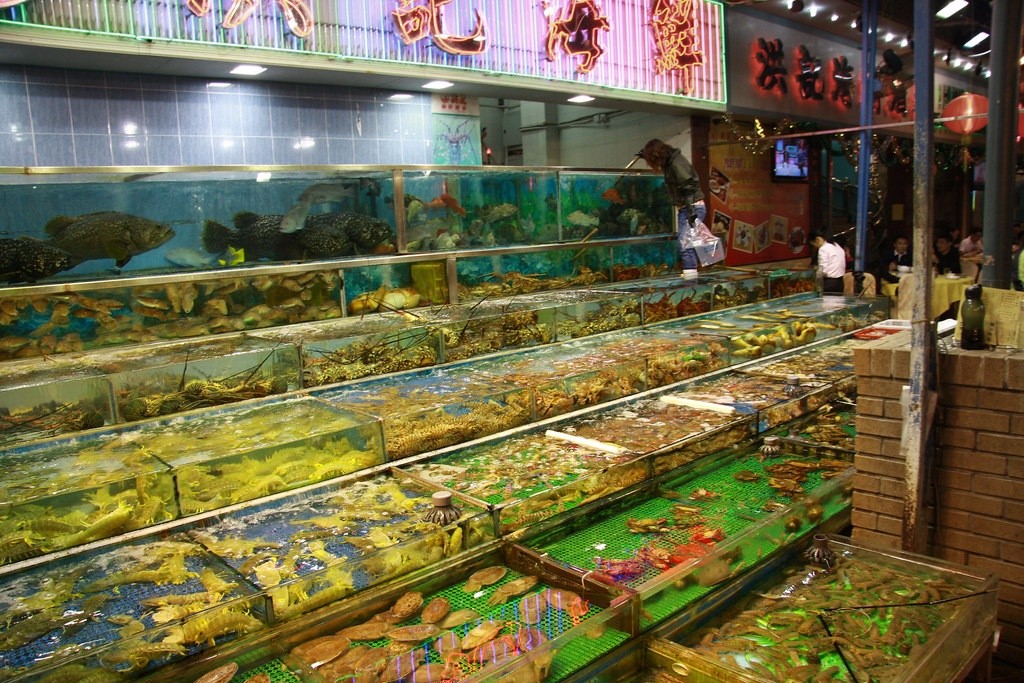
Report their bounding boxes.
[807,227,846,296]
[834,221,1024,294]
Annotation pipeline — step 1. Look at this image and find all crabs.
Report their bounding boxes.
[0,272,819,439]
[458,259,672,299]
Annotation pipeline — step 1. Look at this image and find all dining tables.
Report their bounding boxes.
[880,272,975,322]
[959,251,985,268]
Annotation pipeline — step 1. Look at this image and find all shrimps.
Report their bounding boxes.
[692,556,958,683]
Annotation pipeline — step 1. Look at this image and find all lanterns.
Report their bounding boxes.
[940,92,990,145]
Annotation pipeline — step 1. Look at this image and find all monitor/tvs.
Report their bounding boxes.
[771,139,811,182]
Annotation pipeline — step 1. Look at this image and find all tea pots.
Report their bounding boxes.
[896,265,911,273]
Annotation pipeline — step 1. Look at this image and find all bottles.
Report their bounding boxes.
[960,284,987,350]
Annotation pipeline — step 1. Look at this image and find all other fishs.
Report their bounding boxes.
[0,173,678,285]
[0,259,423,374]
[0,299,887,683]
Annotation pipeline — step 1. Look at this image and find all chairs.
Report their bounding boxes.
[958,261,979,282]
[843,272,876,299]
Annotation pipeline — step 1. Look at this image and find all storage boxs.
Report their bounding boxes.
[0,161,996,683]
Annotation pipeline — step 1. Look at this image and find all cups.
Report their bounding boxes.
[943,268,951,278]
[911,266,914,272]
[932,271,938,280]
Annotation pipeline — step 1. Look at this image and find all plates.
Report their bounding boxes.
[945,275,961,279]
